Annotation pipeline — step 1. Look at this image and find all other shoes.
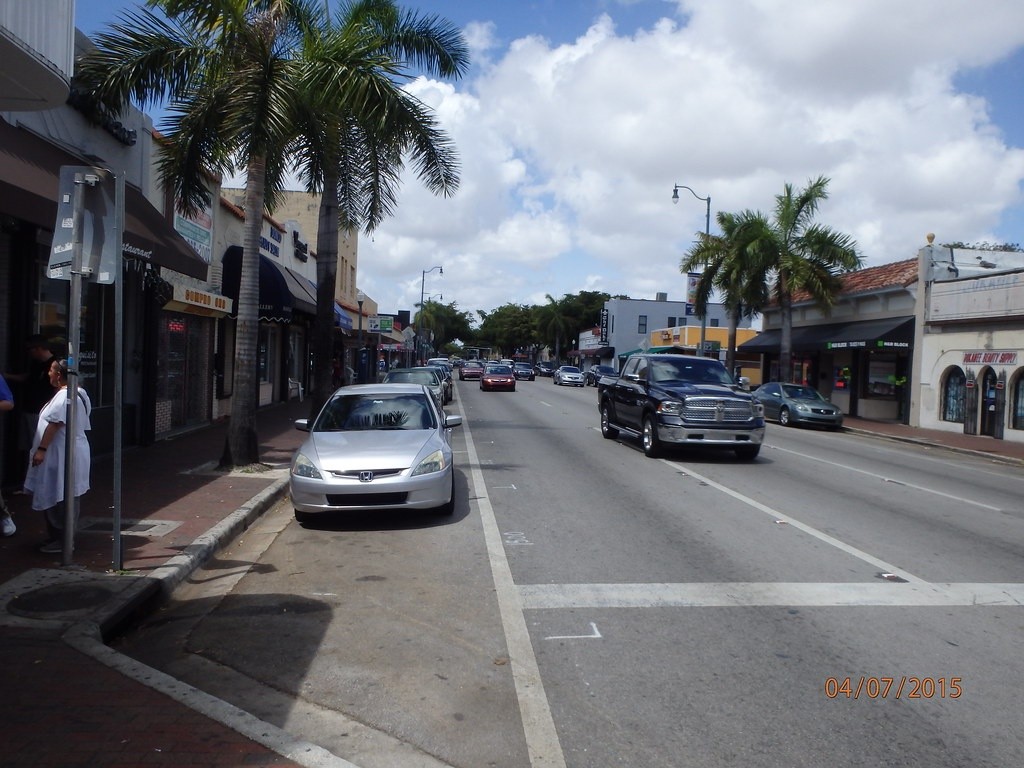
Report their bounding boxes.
[1,507,16,535]
[40,540,75,553]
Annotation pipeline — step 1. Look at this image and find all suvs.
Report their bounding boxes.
[512,362,535,381]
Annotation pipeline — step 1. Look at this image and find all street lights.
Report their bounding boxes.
[425,293,443,363]
[672,181,712,355]
[418,266,443,365]
[356,290,365,384]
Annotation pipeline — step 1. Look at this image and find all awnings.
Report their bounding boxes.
[618,347,682,360]
[222,246,353,330]
[567,347,614,358]
[737,315,915,352]
[0,120,251,282]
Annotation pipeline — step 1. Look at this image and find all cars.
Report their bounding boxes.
[479,364,516,391]
[587,364,619,386]
[459,361,485,381]
[427,358,449,365]
[533,361,556,377]
[380,371,444,412]
[553,365,584,386]
[450,357,463,366]
[411,367,453,405]
[288,385,460,522]
[424,364,453,381]
[484,361,499,365]
[750,382,843,427]
[499,359,515,369]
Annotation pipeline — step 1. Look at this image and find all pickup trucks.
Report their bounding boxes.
[596,356,766,463]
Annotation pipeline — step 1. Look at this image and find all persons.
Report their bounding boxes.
[0,374,17,536]
[7,334,57,452]
[23,359,91,553]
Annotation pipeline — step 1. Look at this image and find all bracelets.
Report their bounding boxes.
[38,447,46,451]
